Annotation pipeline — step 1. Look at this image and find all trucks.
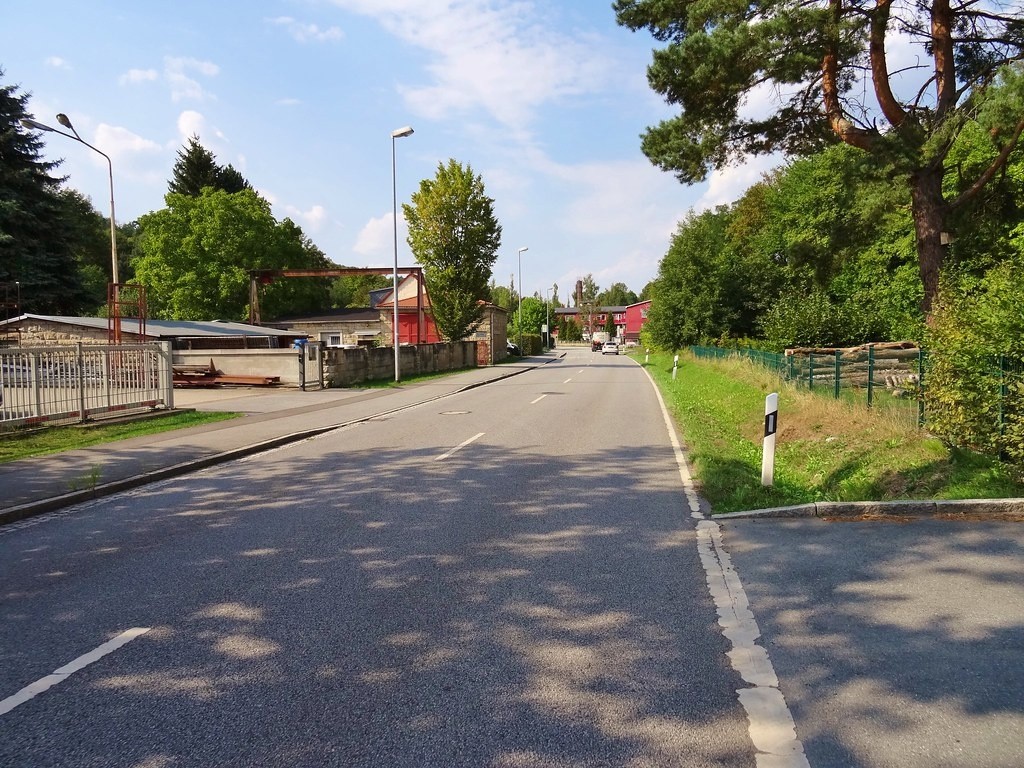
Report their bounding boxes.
[592,332,610,352]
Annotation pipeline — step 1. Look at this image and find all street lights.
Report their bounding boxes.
[587,303,592,344]
[517,248,529,356]
[21,114,124,384]
[392,128,414,381]
[547,287,552,350]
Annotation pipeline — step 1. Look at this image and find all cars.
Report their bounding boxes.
[507,339,519,357]
[601,341,619,356]
